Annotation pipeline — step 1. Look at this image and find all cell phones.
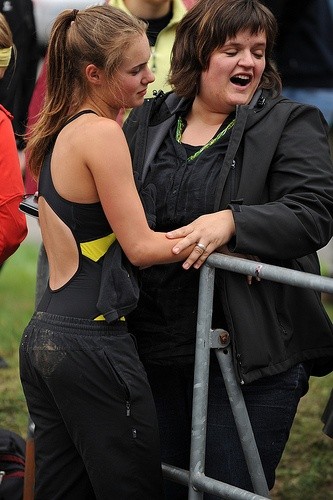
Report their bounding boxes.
[19,195,40,218]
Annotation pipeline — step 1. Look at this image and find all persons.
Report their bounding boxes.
[21,7,200,500]
[122,0,333,500]
[24,0,189,312]
[0,12,28,269]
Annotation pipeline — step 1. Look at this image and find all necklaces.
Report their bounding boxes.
[176,113,236,164]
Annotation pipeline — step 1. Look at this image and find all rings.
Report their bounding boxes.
[197,243,206,250]
